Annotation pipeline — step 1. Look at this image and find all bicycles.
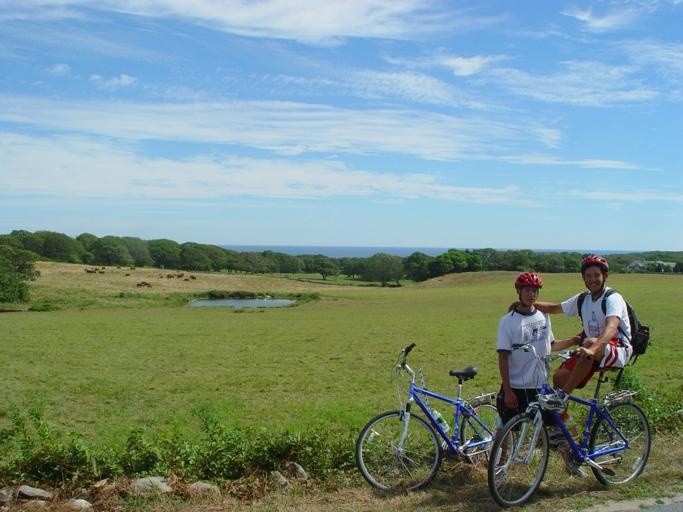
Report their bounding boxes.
[488,344,651,506]
[356,343,514,493]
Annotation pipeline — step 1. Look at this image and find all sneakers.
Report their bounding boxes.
[548,432,580,447]
[566,465,588,478]
[535,387,570,414]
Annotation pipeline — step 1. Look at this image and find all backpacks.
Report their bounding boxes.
[577,289,649,354]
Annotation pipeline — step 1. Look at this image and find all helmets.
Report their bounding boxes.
[515,272,543,288]
[580,256,609,272]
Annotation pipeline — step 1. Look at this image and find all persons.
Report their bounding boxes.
[509,255,634,445]
[497,271,589,479]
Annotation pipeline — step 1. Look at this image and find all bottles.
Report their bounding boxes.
[563,413,579,440]
[636,325,649,355]
[432,409,451,433]
